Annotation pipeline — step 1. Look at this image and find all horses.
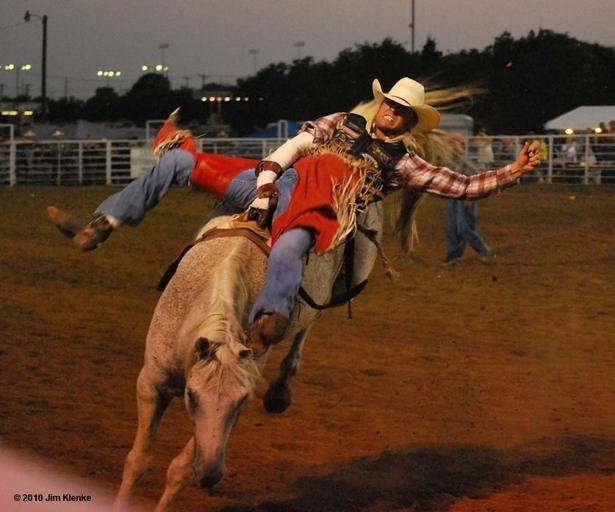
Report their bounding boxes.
[111,83,486,512]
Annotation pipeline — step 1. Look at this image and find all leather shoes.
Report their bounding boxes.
[244,313,287,357]
[46,207,103,252]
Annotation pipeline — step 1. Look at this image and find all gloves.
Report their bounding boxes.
[248,194,273,229]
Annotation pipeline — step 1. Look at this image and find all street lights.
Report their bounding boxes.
[97,70,121,87]
[4,63,32,103]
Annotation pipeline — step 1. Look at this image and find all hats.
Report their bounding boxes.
[372,77,440,134]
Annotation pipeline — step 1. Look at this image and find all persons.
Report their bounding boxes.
[442,135,491,268]
[47,74,543,357]
[476,120,615,185]
